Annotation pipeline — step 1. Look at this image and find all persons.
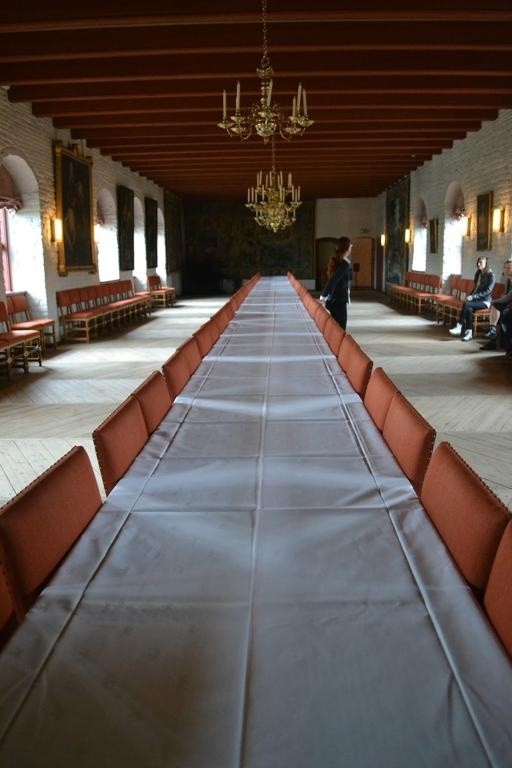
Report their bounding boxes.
[446,255,496,342]
[316,235,355,334]
[478,258,512,360]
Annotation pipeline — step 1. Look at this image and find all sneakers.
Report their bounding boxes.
[484,330,496,337]
[478,342,497,350]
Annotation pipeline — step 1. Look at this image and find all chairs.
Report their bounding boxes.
[55,280,151,343]
[288,270,372,395]
[389,268,507,340]
[162,270,264,398]
[2,369,172,630]
[145,275,177,309]
[1,289,57,379]
[361,366,512,660]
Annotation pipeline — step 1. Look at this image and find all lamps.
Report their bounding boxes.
[492,208,505,233]
[458,215,471,240]
[244,134,303,234]
[217,1,314,144]
[52,214,64,242]
[404,228,410,244]
[380,233,385,248]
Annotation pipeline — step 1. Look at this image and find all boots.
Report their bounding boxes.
[462,328,473,341]
[448,322,462,337]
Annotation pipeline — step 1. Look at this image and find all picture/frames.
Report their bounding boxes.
[52,140,98,276]
[164,189,184,275]
